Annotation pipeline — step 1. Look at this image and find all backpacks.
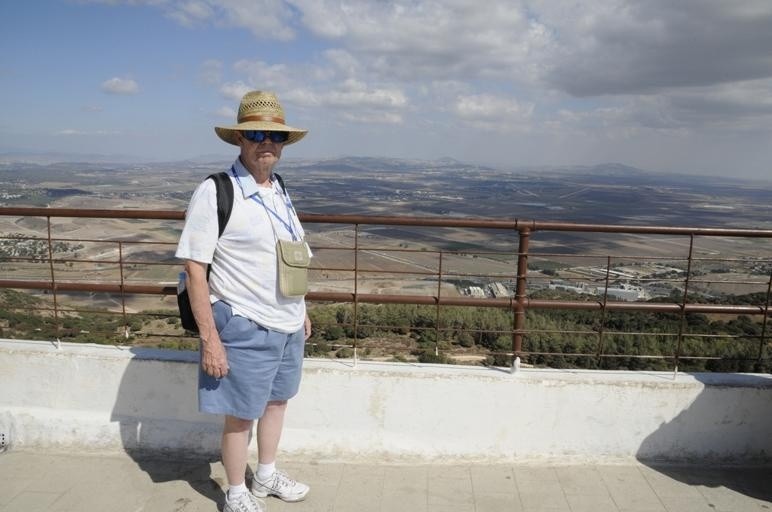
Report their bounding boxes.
[177,172,234,333]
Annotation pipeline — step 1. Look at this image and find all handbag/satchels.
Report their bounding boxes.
[276,239,309,297]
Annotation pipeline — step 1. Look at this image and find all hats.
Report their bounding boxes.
[215,90,308,146]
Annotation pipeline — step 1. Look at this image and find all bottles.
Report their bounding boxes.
[177,272,187,296]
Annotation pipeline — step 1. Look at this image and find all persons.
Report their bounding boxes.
[175,92,313,511]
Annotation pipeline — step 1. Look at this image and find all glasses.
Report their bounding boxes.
[241,130,288,142]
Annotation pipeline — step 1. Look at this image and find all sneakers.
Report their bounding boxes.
[223,470,310,512]
